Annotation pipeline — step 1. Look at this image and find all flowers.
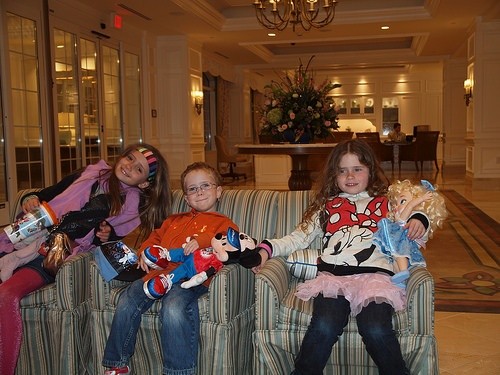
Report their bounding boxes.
[255,55,342,142]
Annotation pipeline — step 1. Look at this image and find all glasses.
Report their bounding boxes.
[185,183,216,193]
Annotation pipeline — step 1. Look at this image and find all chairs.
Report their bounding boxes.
[380,125,440,174]
[214,136,247,180]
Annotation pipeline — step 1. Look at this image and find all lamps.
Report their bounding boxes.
[192,91,204,115]
[254,0,339,32]
[463,79,471,107]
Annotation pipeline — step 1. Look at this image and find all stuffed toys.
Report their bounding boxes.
[141,227,262,300]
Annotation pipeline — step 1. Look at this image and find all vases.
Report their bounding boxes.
[287,130,311,144]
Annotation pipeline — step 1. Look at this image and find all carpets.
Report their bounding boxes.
[420,189,500,314]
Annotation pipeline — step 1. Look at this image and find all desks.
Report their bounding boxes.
[381,144,433,171]
[238,144,337,190]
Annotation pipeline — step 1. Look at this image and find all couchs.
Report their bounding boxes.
[10,187,439,375]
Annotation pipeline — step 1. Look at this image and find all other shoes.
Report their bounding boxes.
[104,365,131,375]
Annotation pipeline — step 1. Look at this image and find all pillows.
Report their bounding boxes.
[286,249,321,279]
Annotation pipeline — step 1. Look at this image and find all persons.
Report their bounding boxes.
[252,138,431,375]
[101,161,239,375]
[388,122,407,142]
[0,142,174,375]
[374,178,448,288]
